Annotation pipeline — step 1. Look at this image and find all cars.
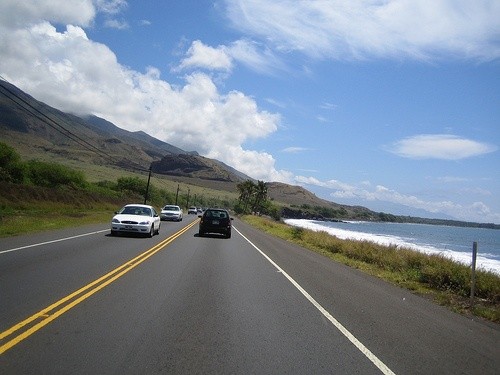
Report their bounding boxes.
[188,206,197,215]
[197,207,202,213]
[111,203,161,238]
[160,205,183,221]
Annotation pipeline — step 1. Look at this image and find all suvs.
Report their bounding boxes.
[198,208,234,239]
